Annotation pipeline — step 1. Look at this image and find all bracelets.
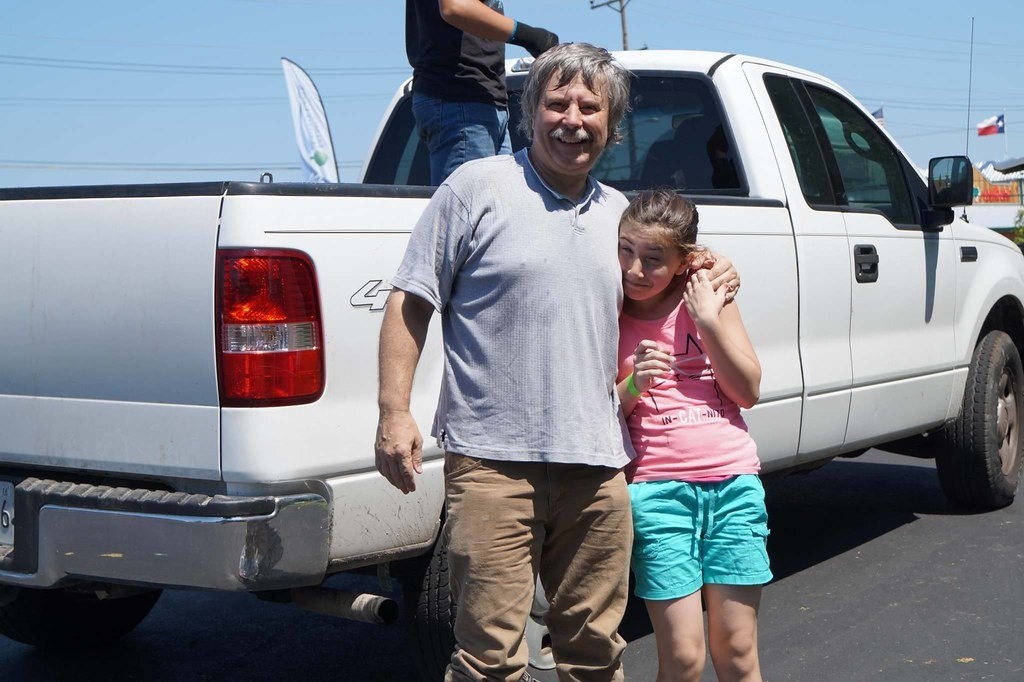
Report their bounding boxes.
[627,371,643,398]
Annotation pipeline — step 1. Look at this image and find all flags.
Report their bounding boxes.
[975,113,1006,137]
[871,108,884,125]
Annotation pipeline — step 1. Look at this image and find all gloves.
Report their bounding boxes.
[506,20,558,60]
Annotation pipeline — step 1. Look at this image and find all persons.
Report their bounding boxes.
[611,187,774,682]
[374,41,742,682]
[405,0,559,187]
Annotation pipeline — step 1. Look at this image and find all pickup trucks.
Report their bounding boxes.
[0,50,1024,669]
